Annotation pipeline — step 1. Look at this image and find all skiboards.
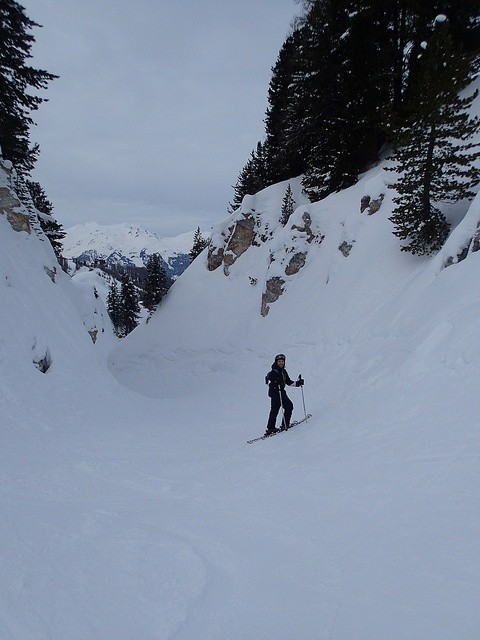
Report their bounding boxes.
[246,413,312,445]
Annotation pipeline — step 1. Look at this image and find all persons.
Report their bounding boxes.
[264,354,304,432]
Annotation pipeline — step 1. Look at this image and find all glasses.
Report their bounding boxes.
[275,355,285,359]
